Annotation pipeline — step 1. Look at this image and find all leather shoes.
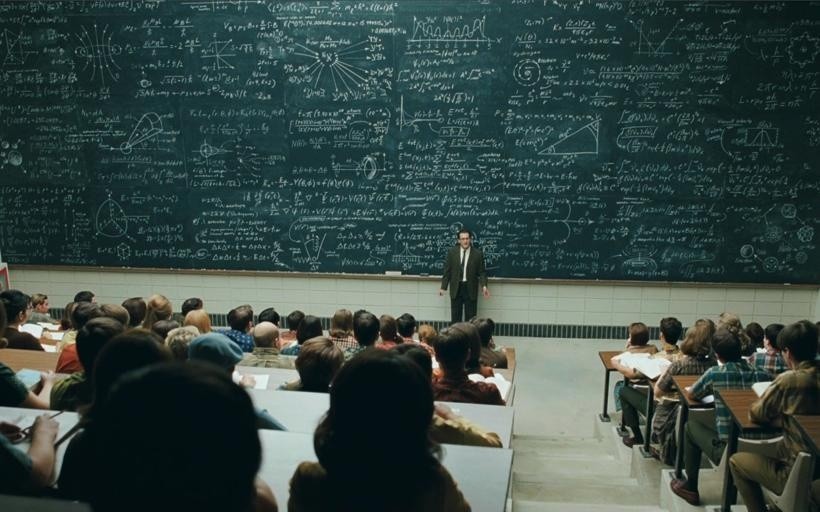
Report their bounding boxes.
[623,435,636,448]
[671,479,699,505]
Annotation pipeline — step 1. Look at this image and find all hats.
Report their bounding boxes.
[188,332,243,369]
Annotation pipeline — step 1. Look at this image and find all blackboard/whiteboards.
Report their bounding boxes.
[0,0,820,290]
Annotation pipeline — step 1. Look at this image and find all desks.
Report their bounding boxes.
[598,350,820,512]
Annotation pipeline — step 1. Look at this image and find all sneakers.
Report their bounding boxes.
[652,432,660,443]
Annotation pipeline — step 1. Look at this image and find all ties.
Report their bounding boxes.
[460,249,466,281]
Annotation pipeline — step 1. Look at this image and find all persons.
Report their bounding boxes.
[614,311,819,512]
[436,230,490,323]
[278,310,508,511]
[1,291,294,512]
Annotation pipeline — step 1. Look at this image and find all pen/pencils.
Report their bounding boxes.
[23,410,64,432]
